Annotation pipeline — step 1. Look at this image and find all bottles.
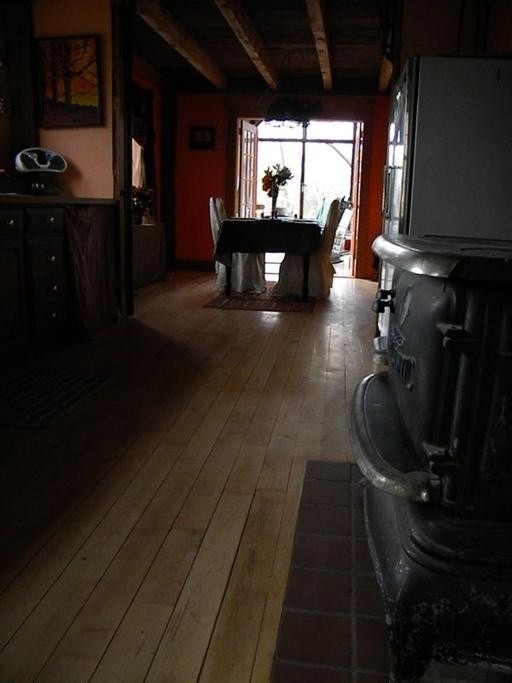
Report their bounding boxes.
[275,184,290,222]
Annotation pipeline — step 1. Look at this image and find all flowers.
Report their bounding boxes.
[132,186,155,213]
[261,163,293,199]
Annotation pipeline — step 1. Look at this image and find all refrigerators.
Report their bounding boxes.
[370,52,512,358]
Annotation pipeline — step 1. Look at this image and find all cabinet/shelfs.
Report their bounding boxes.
[25,210,70,351]
[0,209,23,354]
[69,206,119,338]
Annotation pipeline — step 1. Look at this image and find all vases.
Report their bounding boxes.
[271,191,278,219]
[134,214,143,224]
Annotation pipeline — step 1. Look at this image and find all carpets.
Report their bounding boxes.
[201,281,316,313]
[267,456,390,681]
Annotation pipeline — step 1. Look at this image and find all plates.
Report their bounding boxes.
[292,219,316,222]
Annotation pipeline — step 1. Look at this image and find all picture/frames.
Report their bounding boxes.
[32,35,104,128]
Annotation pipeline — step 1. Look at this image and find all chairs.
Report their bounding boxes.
[208,194,348,302]
